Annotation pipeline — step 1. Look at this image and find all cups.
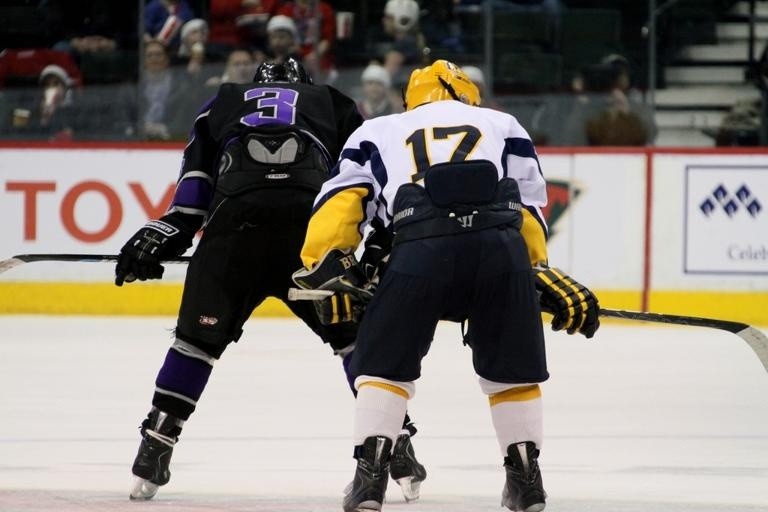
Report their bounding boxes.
[156,15,181,46]
[336,11,356,41]
[43,86,60,110]
[8,110,32,128]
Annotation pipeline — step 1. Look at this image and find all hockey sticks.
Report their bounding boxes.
[285,281,768,368]
[0,250,192,275]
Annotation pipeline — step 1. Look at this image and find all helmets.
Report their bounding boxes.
[406,60,481,111]
[253,57,312,84]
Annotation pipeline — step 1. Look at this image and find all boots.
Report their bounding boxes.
[343,436,393,512]
[390,415,426,485]
[133,404,186,485]
[501,440,545,512]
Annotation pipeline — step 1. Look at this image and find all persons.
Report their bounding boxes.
[287,62,599,512]
[0,0,768,147]
[114,56,426,486]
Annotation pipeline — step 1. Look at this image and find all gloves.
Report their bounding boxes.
[116,211,204,286]
[292,249,380,324]
[527,261,599,338]
